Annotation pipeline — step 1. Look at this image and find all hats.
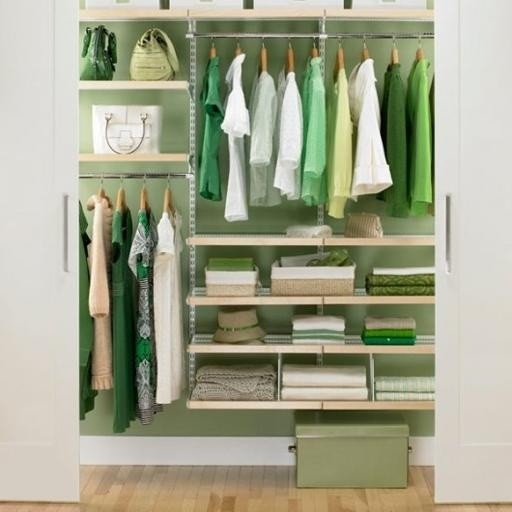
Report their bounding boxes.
[212,307,267,342]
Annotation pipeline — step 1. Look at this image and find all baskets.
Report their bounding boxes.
[270,252,357,296]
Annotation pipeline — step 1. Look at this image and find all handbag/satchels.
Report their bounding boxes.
[130,28,182,81]
[79,25,118,80]
[343,212,383,240]
[287,225,332,239]
[92,105,160,155]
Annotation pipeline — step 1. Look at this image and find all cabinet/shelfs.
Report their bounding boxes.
[79,9,435,437]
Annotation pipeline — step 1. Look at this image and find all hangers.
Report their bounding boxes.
[96,172,176,217]
[210,30,426,76]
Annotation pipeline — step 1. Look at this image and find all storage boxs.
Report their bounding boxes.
[269,261,356,295]
[289,410,413,490]
